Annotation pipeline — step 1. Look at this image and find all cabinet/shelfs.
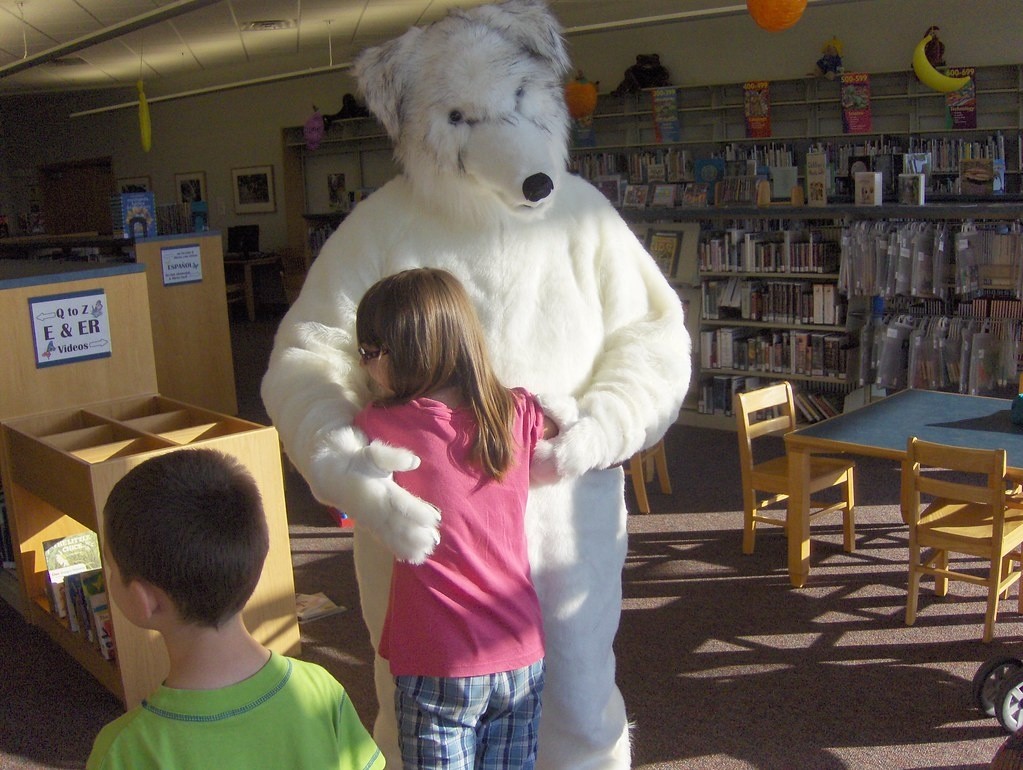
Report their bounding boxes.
[0,392,301,712]
[281,114,401,272]
[570,126,1023,438]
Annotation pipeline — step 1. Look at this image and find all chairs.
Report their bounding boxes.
[735,382,855,554]
[904,436,1023,643]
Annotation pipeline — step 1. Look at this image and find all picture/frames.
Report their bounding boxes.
[231,165,276,215]
[175,170,208,203]
[118,176,152,194]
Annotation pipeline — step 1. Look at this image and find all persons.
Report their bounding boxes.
[84,448,386,770]
[347,269,556,770]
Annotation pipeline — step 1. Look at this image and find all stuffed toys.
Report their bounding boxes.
[611,54,674,102]
[815,38,845,82]
[257,0,693,770]
[911,25,944,80]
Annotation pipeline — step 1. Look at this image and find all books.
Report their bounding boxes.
[43,533,115,661]
[307,221,331,250]
[568,132,1023,425]
[8,191,208,263]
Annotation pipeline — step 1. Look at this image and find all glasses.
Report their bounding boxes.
[357,348,387,364]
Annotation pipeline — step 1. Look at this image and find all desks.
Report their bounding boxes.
[223,256,280,322]
[783,388,1023,588]
